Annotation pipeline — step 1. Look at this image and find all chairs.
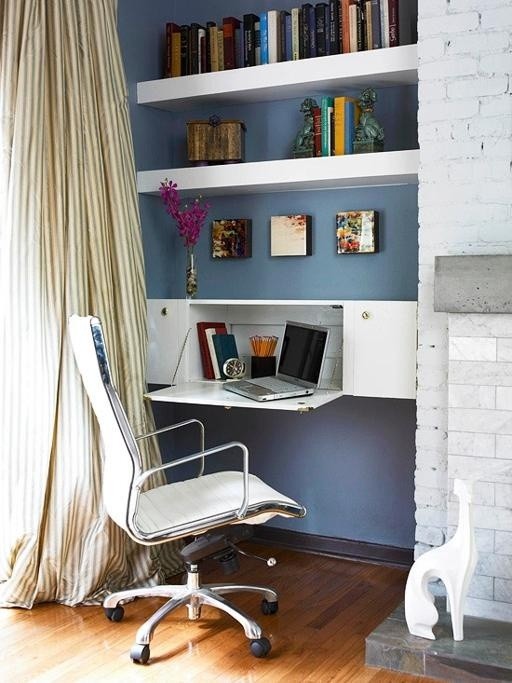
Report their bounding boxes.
[73,314,307,664]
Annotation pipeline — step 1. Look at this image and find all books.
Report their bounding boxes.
[205,327,234,380]
[212,334,243,379]
[314,96,361,158]
[166,0,411,79]
[197,321,233,378]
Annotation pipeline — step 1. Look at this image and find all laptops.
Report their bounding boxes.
[222,320,331,402]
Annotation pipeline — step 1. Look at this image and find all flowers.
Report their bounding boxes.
[159,177,212,248]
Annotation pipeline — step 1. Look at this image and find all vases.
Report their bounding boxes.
[185,254,198,299]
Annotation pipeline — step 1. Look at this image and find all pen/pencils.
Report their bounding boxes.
[249,335,279,357]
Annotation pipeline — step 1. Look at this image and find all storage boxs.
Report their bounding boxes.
[185,121,247,166]
